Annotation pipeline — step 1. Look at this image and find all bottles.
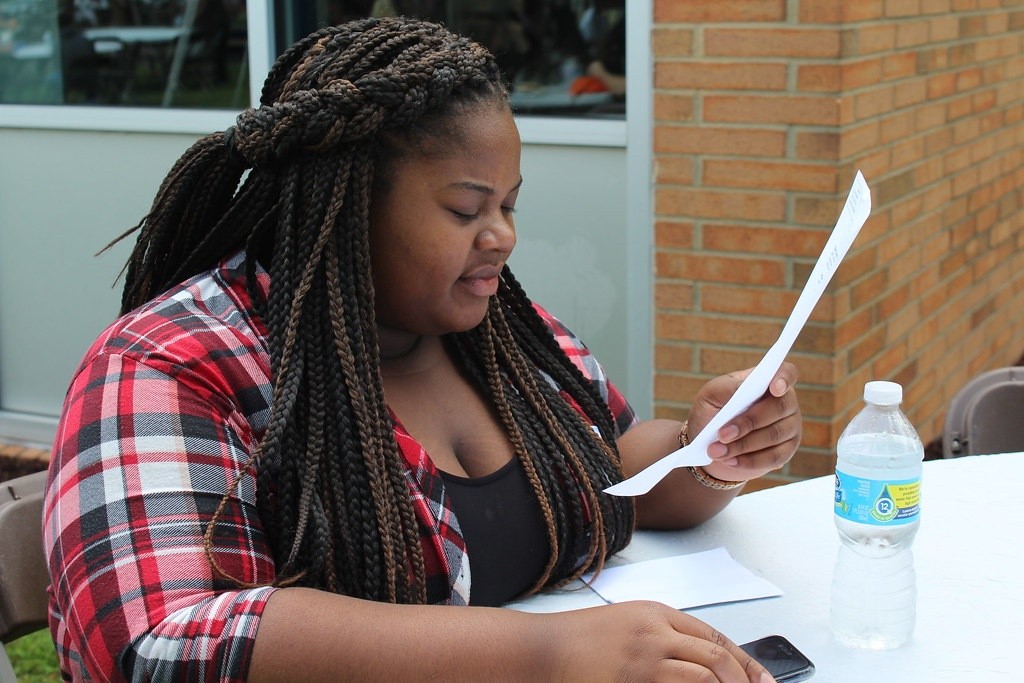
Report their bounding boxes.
[831,381,924,647]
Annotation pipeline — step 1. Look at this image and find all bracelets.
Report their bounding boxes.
[676,417,750,493]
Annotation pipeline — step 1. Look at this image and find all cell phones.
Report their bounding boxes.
[738,634,815,683]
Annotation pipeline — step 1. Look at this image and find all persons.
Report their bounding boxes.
[38,1,807,683]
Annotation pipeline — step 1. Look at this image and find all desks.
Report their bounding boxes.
[495,447,1024,683]
